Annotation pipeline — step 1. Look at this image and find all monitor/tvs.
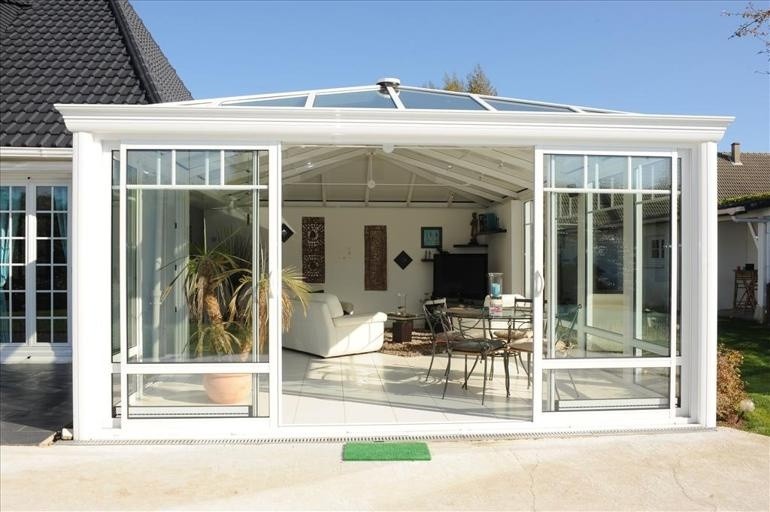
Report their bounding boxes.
[433,252,489,307]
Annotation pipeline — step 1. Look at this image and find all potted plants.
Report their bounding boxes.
[155,225,311,404]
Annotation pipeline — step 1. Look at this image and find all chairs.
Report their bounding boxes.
[423,297,468,390]
[494,297,548,383]
[505,304,582,400]
[440,308,510,405]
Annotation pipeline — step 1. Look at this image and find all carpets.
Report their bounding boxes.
[376,327,448,358]
[343,442,432,461]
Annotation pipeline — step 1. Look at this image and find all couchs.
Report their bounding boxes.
[282,292,388,359]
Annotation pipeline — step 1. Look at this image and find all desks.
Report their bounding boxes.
[442,308,534,396]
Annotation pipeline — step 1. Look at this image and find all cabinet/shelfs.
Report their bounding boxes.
[433,253,488,334]
[421,228,507,262]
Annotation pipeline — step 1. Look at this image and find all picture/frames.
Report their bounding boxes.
[420,226,443,248]
